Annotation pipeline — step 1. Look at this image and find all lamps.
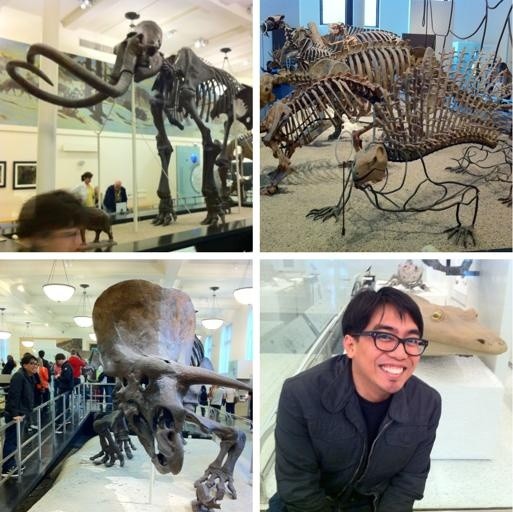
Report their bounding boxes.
[21,322,34,348]
[200,318,224,329]
[233,287,253,306]
[73,284,93,328]
[0,307,11,340]
[43,260,76,303]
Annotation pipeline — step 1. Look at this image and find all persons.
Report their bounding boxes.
[17,190,82,252]
[192,382,253,431]
[494,62,511,101]
[69,172,97,209]
[267,284,441,512]
[102,178,128,214]
[0,350,113,478]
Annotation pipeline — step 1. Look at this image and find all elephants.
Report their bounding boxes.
[77,206,112,244]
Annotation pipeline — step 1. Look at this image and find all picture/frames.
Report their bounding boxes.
[0,160,7,188]
[12,161,37,189]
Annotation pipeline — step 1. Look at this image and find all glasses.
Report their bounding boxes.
[348,331,428,357]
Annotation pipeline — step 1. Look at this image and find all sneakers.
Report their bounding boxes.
[0,464,25,478]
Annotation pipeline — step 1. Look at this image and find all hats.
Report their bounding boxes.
[55,353,65,360]
